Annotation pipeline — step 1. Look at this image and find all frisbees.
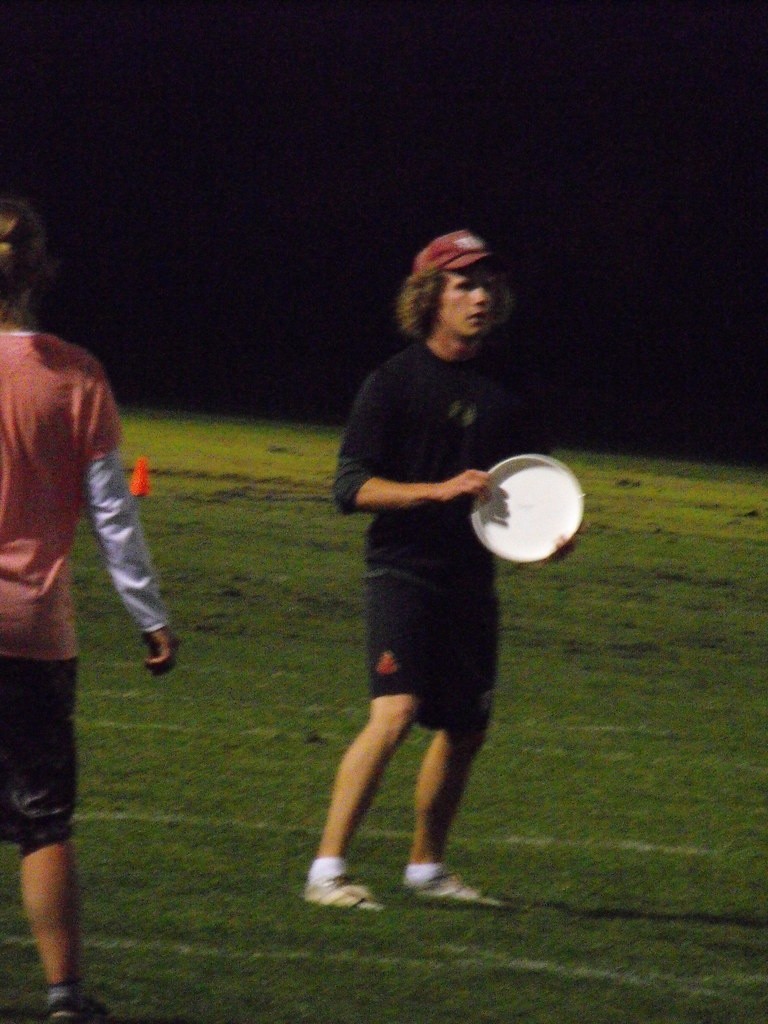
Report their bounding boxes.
[470,453,585,564]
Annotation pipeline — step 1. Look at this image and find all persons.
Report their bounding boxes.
[301,229,588,912]
[0,195,180,1024]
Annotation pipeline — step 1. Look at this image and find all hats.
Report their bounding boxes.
[411,228,493,276]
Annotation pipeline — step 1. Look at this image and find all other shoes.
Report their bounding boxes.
[403,873,492,908]
[303,874,389,913]
[45,983,109,1024]
